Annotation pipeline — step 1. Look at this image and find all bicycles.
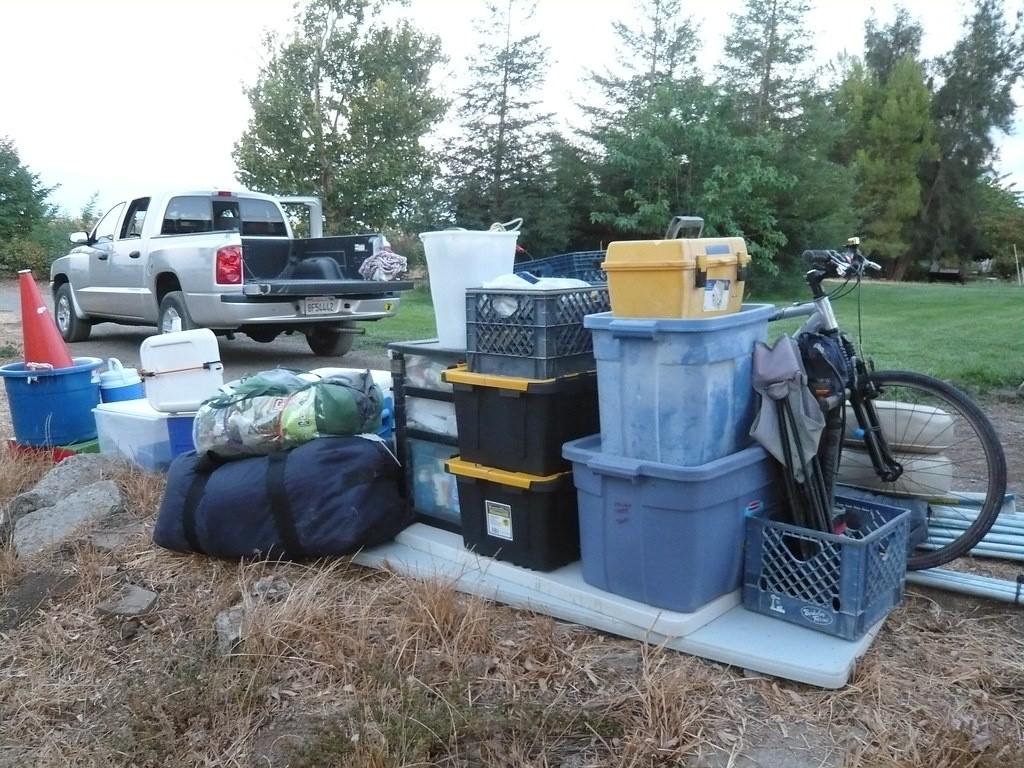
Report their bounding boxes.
[768,237,1008,570]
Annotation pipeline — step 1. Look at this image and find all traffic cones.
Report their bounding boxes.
[18,268,74,372]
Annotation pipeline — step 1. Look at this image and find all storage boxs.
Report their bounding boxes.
[90,315,225,474]
[297,214,911,643]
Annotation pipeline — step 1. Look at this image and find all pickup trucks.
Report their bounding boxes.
[49,182,415,357]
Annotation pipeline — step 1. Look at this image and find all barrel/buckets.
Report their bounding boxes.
[419,229,523,347]
[99,357,144,404]
[0,356,105,448]
